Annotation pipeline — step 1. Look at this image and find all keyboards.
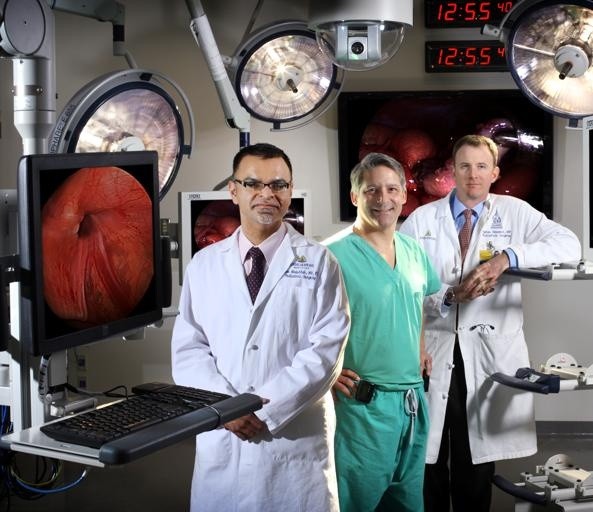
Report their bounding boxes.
[132,383,232,404]
[40,393,205,449]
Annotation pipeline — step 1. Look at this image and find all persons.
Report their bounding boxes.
[317,150,443,512]
[401,135,582,511]
[172,142,352,510]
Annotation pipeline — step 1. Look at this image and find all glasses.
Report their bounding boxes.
[227,176,291,193]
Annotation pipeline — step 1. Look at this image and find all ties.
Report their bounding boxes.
[457,206,475,261]
[243,245,267,305]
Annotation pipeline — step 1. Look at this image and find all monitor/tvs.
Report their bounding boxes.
[17,150,163,356]
[178,189,310,286]
[331,81,566,225]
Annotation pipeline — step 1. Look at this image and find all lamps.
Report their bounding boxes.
[480,0,593,130]
[185,0,337,131]
[48,0,194,204]
